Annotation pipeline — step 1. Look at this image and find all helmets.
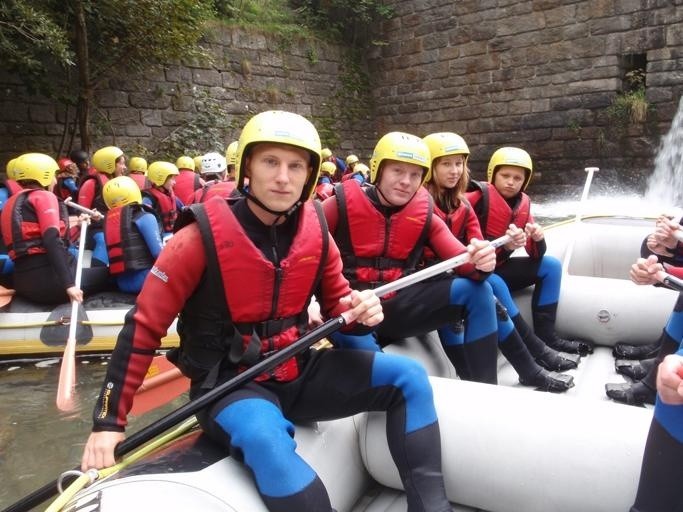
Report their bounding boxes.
[201,152,226,173]
[70,149,89,164]
[93,146,123,174]
[6,158,17,180]
[322,148,332,158]
[353,164,369,174]
[103,176,142,208]
[346,155,359,165]
[176,156,195,171]
[16,153,60,187]
[423,132,470,182]
[487,147,533,191]
[148,161,179,187]
[322,162,336,175]
[58,159,73,170]
[194,156,203,170]
[129,157,147,173]
[370,132,430,184]
[226,141,239,165]
[235,110,322,202]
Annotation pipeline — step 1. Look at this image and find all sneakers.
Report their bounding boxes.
[518,370,574,392]
[536,349,577,371]
[612,342,654,359]
[606,382,655,405]
[615,359,652,379]
[546,338,593,356]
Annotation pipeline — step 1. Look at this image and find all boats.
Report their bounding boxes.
[30,206,683,511]
[0,289,195,362]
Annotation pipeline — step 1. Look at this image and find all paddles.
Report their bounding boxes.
[57,220,89,417]
[0,286,16,308]
[129,251,476,417]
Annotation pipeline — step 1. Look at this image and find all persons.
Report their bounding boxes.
[81,109,452,512]
[419,132,576,393]
[464,145,593,372]
[306,131,498,386]
[604,211,683,512]
[1,141,239,306]
[311,145,370,201]
